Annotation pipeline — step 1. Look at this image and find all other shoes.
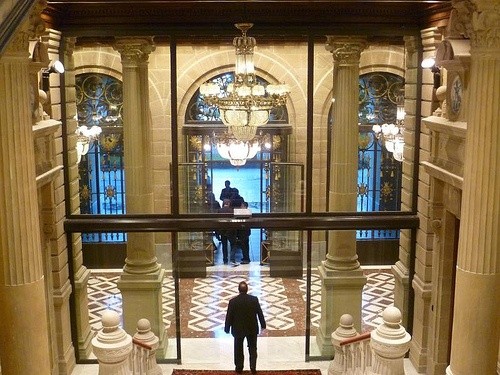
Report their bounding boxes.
[251,369,256,373]
[235,368,243,372]
[230,261,239,265]
[241,260,249,264]
[241,258,250,261]
[224,261,228,264]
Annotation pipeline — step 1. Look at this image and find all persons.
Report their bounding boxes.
[211,180,251,265]
[224,281,266,374]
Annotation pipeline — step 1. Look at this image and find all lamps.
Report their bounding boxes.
[75,125,102,164]
[47,60,65,76]
[372,46,406,162]
[200,23,291,172]
